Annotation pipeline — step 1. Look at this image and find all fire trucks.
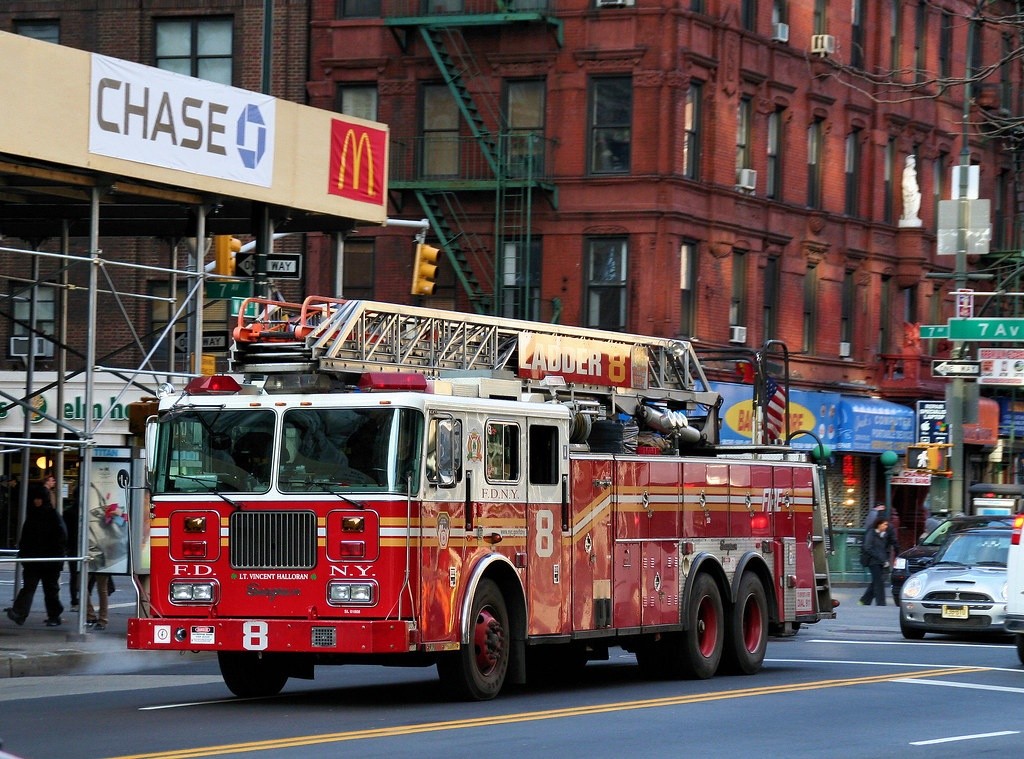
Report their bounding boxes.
[125,296,841,703]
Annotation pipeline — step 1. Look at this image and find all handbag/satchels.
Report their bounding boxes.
[859,529,876,568]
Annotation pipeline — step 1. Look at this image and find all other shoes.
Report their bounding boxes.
[87,619,98,628]
[7,607,26,626]
[47,617,61,626]
[95,624,104,630]
[70,605,80,611]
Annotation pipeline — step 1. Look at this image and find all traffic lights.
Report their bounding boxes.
[228,235,241,275]
[411,245,441,297]
[906,447,929,470]
[930,448,948,472]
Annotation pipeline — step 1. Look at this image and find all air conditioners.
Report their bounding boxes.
[735,167,758,191]
[729,327,748,344]
[810,34,835,53]
[772,23,787,42]
[10,336,53,356]
[839,341,851,356]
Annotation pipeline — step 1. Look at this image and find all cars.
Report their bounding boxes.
[1007,500,1024,663]
[899,528,1016,642]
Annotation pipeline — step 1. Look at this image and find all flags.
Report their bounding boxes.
[759,374,787,442]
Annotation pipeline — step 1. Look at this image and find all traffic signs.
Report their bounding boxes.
[931,359,981,377]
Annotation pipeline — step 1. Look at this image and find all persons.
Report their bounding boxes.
[859,518,888,606]
[63,487,80,605]
[87,573,110,631]
[0,467,57,556]
[864,503,900,579]
[7,486,67,626]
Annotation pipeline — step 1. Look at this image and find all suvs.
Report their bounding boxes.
[891,513,1017,607]
